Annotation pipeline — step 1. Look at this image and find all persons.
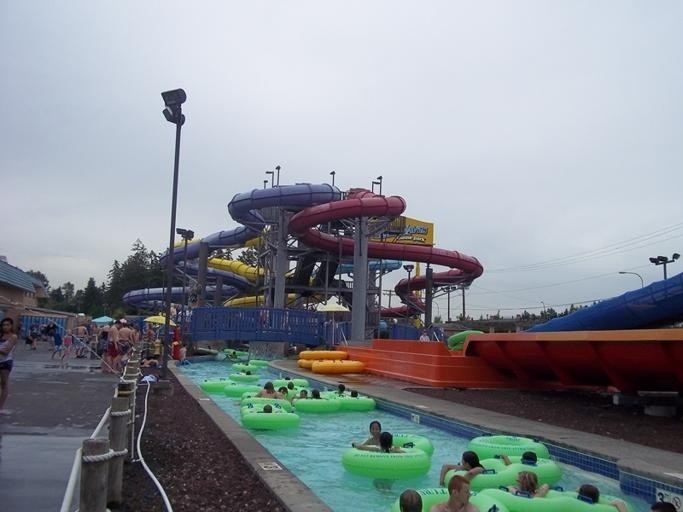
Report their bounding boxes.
[419,329,430,341]
[357,421,406,453]
[400,489,423,511]
[440,450,487,486]
[0,303,193,408]
[507,471,550,499]
[254,376,357,413]
[576,485,625,511]
[431,474,478,511]
[501,452,538,467]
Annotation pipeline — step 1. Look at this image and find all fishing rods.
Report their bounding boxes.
[200,348,377,430]
[343,434,640,512]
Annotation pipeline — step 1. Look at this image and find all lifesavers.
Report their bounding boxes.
[448,330,485,351]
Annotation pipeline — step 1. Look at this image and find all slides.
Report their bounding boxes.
[122,185,484,319]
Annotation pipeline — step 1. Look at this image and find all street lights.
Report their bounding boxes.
[404,265,414,325]
[161,88,194,379]
[619,271,643,288]
[649,253,680,280]
